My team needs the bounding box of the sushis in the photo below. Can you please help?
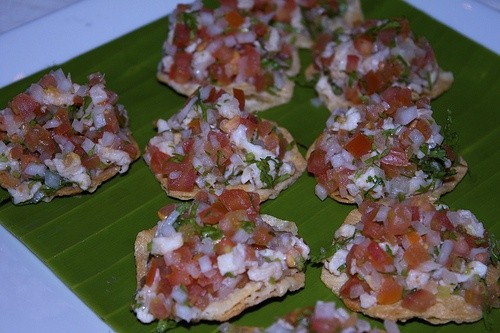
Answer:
[0,0,500,333]
